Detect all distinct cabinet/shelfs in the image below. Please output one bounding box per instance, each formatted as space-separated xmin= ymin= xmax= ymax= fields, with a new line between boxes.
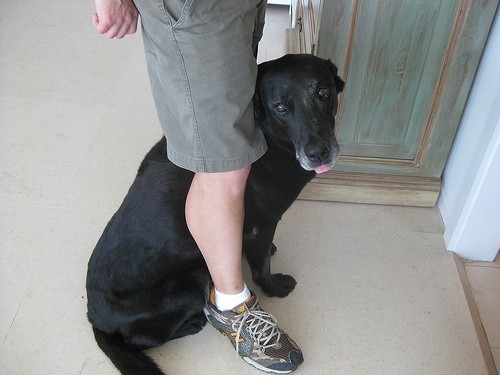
xmin=283 ymin=0 xmax=500 ymax=210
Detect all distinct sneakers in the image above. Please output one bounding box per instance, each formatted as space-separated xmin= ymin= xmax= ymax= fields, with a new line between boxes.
xmin=204 ymin=286 xmax=305 ymax=374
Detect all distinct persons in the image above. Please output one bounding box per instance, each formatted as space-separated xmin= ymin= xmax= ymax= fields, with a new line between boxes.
xmin=92 ymin=0 xmax=304 ymax=374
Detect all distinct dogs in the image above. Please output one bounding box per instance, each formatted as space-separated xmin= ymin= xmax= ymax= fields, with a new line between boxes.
xmin=85 ymin=53 xmax=347 ymax=375
